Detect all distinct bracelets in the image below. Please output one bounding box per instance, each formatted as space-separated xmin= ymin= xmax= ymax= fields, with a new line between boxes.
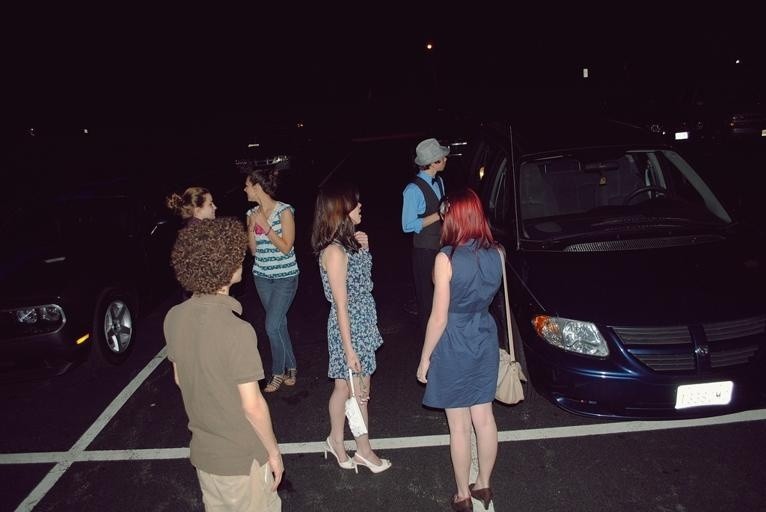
xmin=264 ymin=226 xmax=272 ymax=236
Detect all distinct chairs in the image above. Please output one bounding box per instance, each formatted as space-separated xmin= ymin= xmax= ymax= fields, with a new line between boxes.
xmin=519 ymin=154 xmax=650 ymax=221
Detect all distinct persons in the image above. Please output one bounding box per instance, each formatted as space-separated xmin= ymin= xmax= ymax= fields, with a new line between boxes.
xmin=160 ymin=187 xmax=218 ymax=303
xmin=243 ymin=170 xmax=301 ymax=394
xmin=162 ymin=218 xmax=285 ymax=512
xmin=401 ymin=137 xmax=451 ymax=366
xmin=310 ymin=177 xmax=392 ymax=476
xmin=415 ymin=188 xmax=507 ymax=512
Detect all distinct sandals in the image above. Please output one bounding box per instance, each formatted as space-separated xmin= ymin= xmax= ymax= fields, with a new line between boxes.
xmin=263 ymin=374 xmax=286 ymax=392
xmin=285 ymin=369 xmax=297 ymax=384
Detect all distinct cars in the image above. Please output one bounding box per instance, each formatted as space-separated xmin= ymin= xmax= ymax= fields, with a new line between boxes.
xmin=702 ymin=84 xmax=766 ymax=139
xmin=226 ymin=108 xmax=310 ymax=182
xmin=469 ymin=123 xmax=766 ymax=422
xmin=1 ymin=191 xmax=174 ymax=368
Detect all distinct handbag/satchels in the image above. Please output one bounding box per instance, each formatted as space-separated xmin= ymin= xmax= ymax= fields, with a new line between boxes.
xmin=495 ymin=349 xmax=527 ymax=404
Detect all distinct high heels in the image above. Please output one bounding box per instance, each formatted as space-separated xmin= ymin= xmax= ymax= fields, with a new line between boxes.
xmin=450 ymin=492 xmax=473 ymax=511
xmin=469 ymin=483 xmax=494 ymax=510
xmin=321 ymin=436 xmax=357 ymax=471
xmin=352 ymin=449 xmax=392 ymax=474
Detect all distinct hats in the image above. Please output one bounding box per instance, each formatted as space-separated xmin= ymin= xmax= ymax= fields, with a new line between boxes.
xmin=414 ymin=138 xmax=451 ymax=167
